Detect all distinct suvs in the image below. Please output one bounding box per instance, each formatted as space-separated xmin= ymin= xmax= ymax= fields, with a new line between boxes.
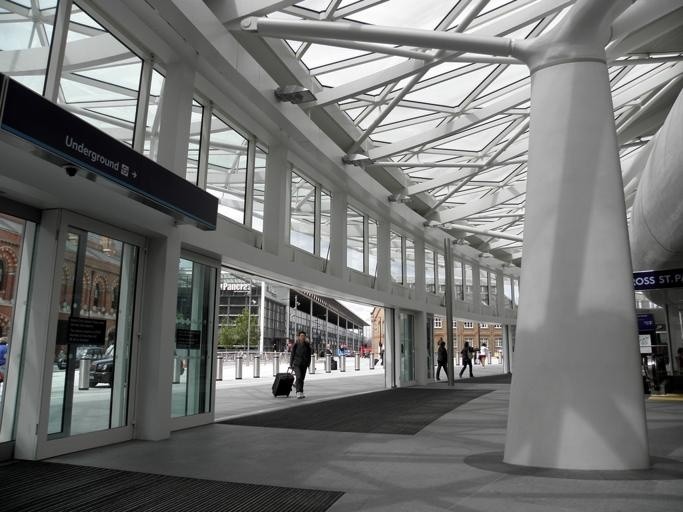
xmin=89 ymin=344 xmax=116 ymax=387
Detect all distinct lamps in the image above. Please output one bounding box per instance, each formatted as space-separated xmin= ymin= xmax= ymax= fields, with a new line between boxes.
xmin=340 ymin=154 xmax=373 ymax=168
xmin=502 ymin=261 xmax=515 ymax=268
xmin=423 ymin=220 xmax=443 ymax=229
xmin=387 ymin=192 xmax=412 ymax=206
xmin=452 ymin=238 xmax=471 ymax=246
xmin=477 ymin=252 xmax=495 ymax=262
xmin=271 ymin=83 xmax=317 ymax=107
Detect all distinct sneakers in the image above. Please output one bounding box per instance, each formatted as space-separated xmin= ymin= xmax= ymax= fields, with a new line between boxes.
xmin=435 ymin=377 xmax=441 ymax=381
xmin=459 ymin=373 xmax=462 ymax=378
xmin=469 ymin=374 xmax=474 ymax=378
xmin=296 ymin=392 xmax=306 ymax=399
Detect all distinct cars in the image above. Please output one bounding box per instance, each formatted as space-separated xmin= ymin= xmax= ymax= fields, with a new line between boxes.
xmin=345 ymin=349 xmax=355 ymax=356
xmin=494 ymin=347 xmax=503 ymax=358
xmin=56 ymin=345 xmax=106 ymax=370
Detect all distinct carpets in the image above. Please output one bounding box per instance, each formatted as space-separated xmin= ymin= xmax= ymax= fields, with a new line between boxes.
xmin=437 ymin=372 xmax=513 ymax=386
xmin=210 ymin=386 xmax=492 ymax=437
xmin=0 ymin=458 xmax=346 ymax=512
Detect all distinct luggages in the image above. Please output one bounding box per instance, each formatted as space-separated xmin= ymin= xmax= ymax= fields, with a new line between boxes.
xmin=324 ymin=360 xmax=337 ymax=370
xmin=372 ymin=359 xmax=379 ymax=365
xmin=271 ymin=372 xmax=294 ymax=398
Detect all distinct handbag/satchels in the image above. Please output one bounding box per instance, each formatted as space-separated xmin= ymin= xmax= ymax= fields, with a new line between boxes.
xmin=468 ymin=347 xmax=473 ymax=360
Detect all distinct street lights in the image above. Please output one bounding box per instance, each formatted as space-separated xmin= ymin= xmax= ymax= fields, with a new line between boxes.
xmin=247 ymin=298 xmax=257 ymax=357
xmin=370 ymin=315 xmax=381 ymax=355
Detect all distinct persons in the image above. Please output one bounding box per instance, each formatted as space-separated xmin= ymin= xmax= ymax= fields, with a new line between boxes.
xmin=435 ymin=337 xmax=490 ymax=381
xmin=57 ymin=349 xmax=66 ymax=359
xmin=0 ymin=336 xmax=8 ymax=383
xmin=675 ymin=348 xmax=683 ymax=378
xmin=323 ymin=341 xmax=387 ymax=366
xmin=273 ymin=340 xmax=294 ymax=353
xmin=289 ymin=331 xmax=311 ymax=399
xmin=642 ymin=356 xmax=652 ymax=394
